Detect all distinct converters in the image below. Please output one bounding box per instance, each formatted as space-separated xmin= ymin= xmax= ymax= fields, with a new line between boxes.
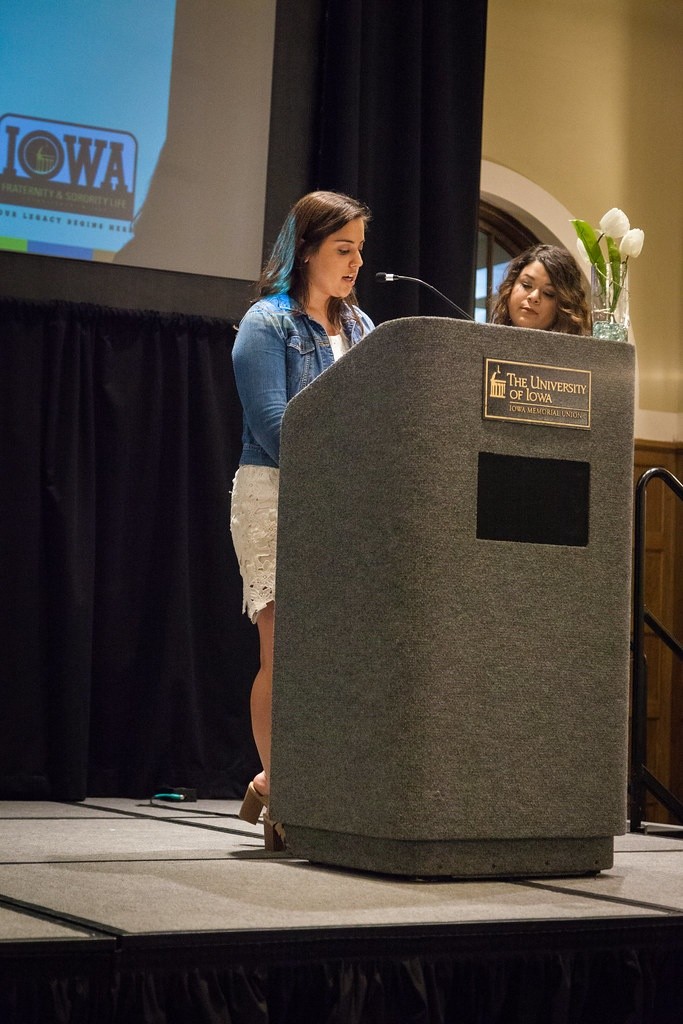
xmin=158 ymin=786 xmax=197 ymax=802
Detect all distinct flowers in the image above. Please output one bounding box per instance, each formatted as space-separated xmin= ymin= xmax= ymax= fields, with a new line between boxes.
xmin=569 ymin=208 xmax=645 ymax=319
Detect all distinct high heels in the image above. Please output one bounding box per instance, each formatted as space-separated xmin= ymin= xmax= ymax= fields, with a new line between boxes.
xmin=263 ymin=813 xmax=283 ymax=852
xmin=238 ymin=781 xmax=269 ymax=825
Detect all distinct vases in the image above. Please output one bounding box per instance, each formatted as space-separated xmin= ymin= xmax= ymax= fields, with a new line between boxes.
xmin=590 ymin=262 xmax=630 ymax=342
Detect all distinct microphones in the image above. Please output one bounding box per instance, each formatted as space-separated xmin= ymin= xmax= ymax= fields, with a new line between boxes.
xmin=376 ymin=273 xmax=475 ymax=321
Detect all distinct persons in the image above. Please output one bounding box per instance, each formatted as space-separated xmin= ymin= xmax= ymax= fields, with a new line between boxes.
xmin=490 ymin=243 xmax=593 ymax=338
xmin=230 ymin=190 xmax=374 ymax=855
